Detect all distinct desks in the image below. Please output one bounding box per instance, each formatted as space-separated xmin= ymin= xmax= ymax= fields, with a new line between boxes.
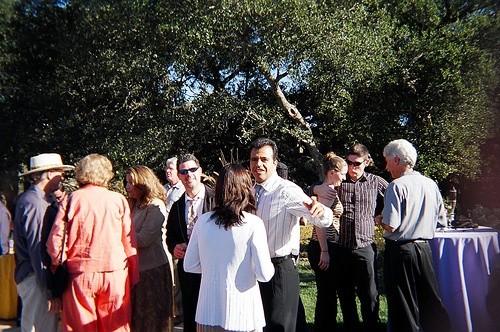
xmin=431 ymin=226 xmax=500 ymax=332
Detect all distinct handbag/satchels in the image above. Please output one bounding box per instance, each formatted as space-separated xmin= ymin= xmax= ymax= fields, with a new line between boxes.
xmin=46 ymin=265 xmax=70 ymax=296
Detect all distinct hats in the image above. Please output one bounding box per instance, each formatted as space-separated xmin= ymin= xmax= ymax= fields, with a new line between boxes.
xmin=19 ymin=154 xmax=75 ymax=176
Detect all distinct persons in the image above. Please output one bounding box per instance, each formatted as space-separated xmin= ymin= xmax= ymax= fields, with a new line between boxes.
xmin=304 ymin=143 xmax=390 ymax=332
xmin=307 ymin=152 xmax=348 ymax=332
xmin=0 ymin=138 xmax=333 ymax=332
xmin=375 ymin=138 xmax=450 ymax=332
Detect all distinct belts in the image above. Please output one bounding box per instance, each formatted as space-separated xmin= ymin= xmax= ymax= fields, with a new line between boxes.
xmin=272 ymin=254 xmax=293 ymax=265
xmin=405 ymin=239 xmax=427 ymax=243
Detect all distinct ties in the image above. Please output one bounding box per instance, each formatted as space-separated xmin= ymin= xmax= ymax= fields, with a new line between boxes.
xmin=187 ymin=200 xmax=198 ymax=240
xmin=254 ymin=184 xmax=263 ymax=210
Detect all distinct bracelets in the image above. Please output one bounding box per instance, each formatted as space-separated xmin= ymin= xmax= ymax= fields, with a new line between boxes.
xmin=321 ymin=249 xmax=328 ymax=252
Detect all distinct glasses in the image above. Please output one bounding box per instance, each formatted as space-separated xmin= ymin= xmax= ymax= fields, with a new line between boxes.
xmin=346 ymin=159 xmax=365 ymax=166
xmin=178 ymin=166 xmax=200 ymax=175
